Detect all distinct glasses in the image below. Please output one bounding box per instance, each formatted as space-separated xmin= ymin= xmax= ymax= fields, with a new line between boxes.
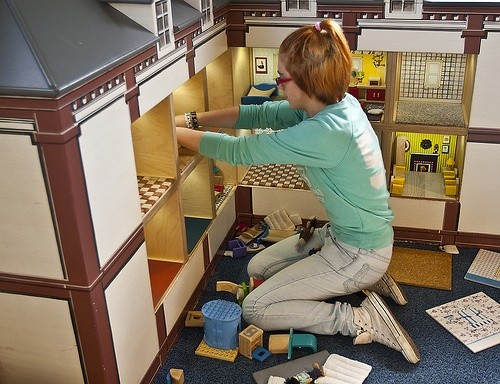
xmin=276 ymin=76 xmax=292 ymax=86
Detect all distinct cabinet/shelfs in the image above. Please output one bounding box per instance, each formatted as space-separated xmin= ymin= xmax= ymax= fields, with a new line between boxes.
xmin=347 ymin=87 xmax=359 ymax=101
xmin=366 ymin=88 xmax=385 ymax=102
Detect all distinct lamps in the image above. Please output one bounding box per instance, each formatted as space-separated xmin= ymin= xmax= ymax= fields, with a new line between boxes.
xmin=446 ymin=157 xmax=455 ymax=171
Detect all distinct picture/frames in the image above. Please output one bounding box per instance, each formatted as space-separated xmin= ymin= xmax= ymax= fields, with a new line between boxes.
xmin=442 ymin=135 xmax=451 ymax=144
xmin=442 ymin=145 xmax=449 ymax=154
xmin=351 ymin=56 xmax=363 ymax=73
xmin=255 ymin=57 xmax=268 ymax=74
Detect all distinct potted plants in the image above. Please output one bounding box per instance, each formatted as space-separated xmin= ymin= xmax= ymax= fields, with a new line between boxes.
xmin=355 ymin=70 xmax=364 ymax=83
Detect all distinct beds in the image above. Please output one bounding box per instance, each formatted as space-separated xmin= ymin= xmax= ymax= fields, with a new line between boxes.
xmin=241 ymin=82 xmax=279 ymax=106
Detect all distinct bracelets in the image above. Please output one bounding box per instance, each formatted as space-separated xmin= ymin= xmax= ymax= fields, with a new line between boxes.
xmin=184 ymin=112 xmax=198 ymax=129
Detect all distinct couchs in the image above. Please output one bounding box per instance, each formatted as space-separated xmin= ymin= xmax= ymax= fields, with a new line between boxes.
xmin=444 ymin=180 xmax=458 ymax=196
xmin=444 ymin=170 xmax=456 ymax=186
xmin=394 ymin=166 xmax=406 ymax=180
xmin=390 ymin=179 xmax=404 ymax=195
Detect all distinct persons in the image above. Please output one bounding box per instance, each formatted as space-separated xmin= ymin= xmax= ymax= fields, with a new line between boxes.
xmin=174 ymin=19 xmax=420 ymax=363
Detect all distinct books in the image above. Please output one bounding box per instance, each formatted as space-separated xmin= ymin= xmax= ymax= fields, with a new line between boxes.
xmin=426 ymin=292 xmax=500 ymax=353
xmin=464 ymin=249 xmax=500 ymax=289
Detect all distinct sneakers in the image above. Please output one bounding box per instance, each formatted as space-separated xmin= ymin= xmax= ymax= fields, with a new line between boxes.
xmin=362 ymin=271 xmax=408 ymax=305
xmin=352 ymin=292 xmax=421 ymax=363
